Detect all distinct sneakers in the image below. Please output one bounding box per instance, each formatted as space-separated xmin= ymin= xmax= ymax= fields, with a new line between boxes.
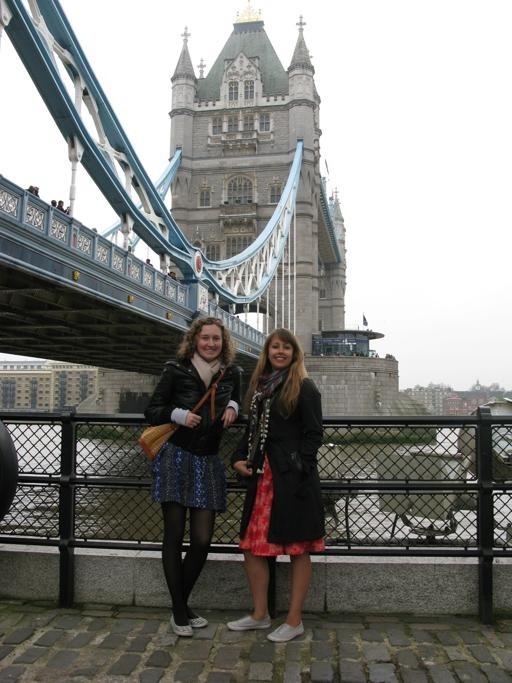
xmin=267 ymin=621 xmax=305 ymax=643
xmin=227 ymin=615 xmax=271 ymax=632
xmin=188 ymin=615 xmax=209 ymax=628
xmin=169 ymin=613 xmax=194 ymax=636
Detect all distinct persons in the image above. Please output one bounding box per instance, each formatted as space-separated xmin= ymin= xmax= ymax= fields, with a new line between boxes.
xmin=168 ymin=271 xmax=177 ymax=280
xmin=230 ymin=328 xmax=326 ymax=642
xmin=144 ymin=316 xmax=244 ymax=638
xmin=145 ymin=258 xmax=153 ymax=266
xmin=333 ymin=349 xmax=392 ymax=358
xmin=25 ymin=185 xmax=70 ymax=214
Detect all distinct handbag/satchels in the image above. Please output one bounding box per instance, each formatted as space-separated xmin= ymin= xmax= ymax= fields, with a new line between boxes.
xmin=137 ymin=421 xmax=177 ymax=460
